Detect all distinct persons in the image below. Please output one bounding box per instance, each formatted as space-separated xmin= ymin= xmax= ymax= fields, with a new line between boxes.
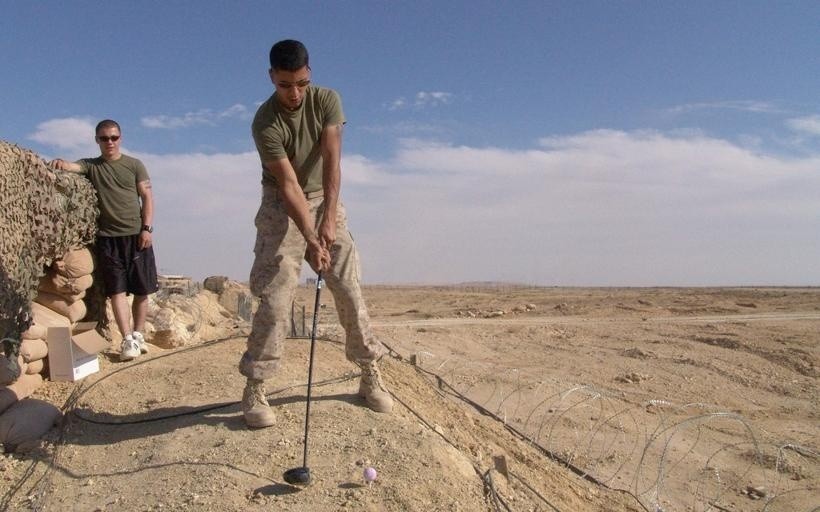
xmin=45 ymin=118 xmax=159 ymax=362
xmin=238 ymin=39 xmax=396 ymax=428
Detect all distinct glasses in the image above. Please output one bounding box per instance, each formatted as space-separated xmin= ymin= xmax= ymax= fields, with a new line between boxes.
xmin=270 ymin=65 xmax=312 ymax=88
xmin=97 ymin=135 xmax=120 ymax=142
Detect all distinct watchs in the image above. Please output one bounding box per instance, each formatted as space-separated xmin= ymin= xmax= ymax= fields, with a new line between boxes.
xmin=141 ymin=224 xmax=153 ymax=233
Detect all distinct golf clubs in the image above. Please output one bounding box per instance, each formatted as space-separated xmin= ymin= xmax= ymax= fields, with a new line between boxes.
xmin=284 ymin=270 xmax=322 ymax=487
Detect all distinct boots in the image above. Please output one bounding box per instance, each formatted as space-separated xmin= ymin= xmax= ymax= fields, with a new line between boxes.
xmin=242 ymin=380 xmax=276 ymax=429
xmin=359 ymin=362 xmax=394 ymax=413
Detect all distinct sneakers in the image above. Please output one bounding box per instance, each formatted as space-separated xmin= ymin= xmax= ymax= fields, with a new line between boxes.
xmin=132 ymin=331 xmax=148 ymax=353
xmin=119 ymin=336 xmax=141 ymax=362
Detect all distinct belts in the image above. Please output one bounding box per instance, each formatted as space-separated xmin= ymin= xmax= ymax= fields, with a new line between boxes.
xmin=263 ymin=184 xmax=325 ymax=201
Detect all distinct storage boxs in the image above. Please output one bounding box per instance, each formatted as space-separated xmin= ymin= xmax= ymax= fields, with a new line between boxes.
xmin=46 ymin=320 xmax=111 ymax=382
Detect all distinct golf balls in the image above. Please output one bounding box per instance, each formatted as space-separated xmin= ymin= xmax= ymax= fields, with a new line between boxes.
xmin=364 ymin=468 xmax=376 ymax=480
